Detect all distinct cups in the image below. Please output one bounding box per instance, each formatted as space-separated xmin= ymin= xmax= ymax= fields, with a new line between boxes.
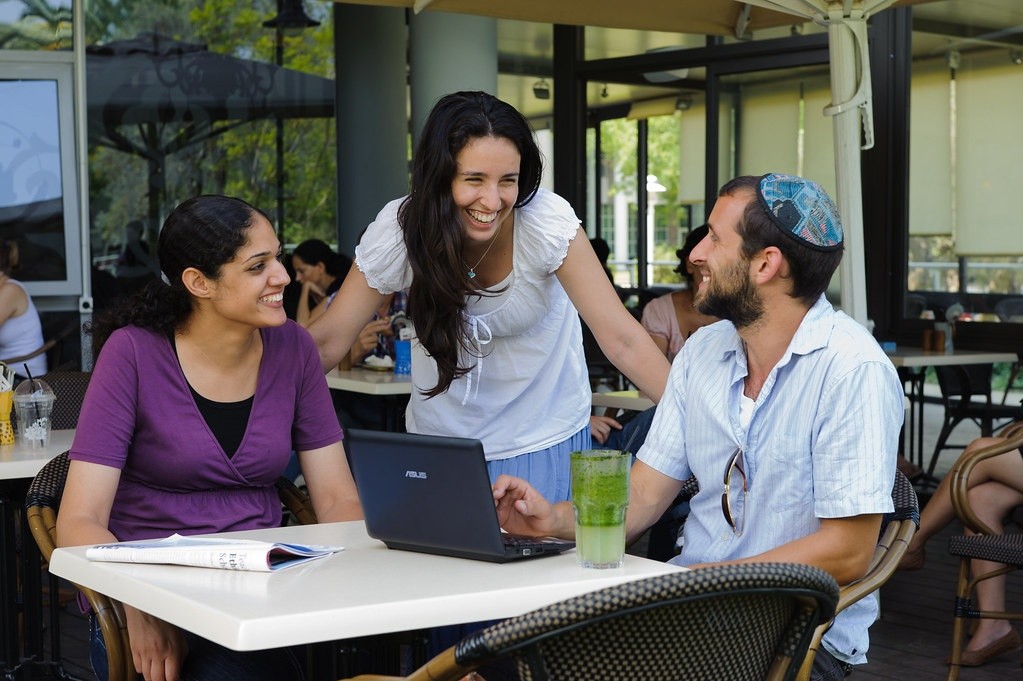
xmin=571 ymin=449 xmax=630 ymax=571
xmin=11 ymin=379 xmax=55 ymax=452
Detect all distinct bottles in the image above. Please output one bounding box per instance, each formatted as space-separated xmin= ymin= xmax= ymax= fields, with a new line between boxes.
xmin=0 ymin=391 xmax=16 ymax=446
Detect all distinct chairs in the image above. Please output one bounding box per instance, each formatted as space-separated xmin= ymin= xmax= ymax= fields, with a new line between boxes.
xmin=0 ymin=286 xmax=1023 ymax=681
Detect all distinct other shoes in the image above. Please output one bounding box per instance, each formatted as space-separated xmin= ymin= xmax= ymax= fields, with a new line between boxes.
xmin=896 ymin=547 xmax=926 ymax=570
xmin=945 ymin=628 xmax=1021 ymax=666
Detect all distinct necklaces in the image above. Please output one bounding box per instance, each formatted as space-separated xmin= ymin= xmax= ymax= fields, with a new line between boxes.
xmin=463 ymin=226 xmax=501 ymax=279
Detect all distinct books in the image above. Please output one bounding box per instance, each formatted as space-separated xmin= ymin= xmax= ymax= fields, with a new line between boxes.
xmin=86 ymin=534 xmax=344 ymax=573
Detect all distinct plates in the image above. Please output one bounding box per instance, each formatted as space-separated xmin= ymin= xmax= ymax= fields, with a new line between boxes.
xmin=357 ymin=362 xmax=395 ymax=371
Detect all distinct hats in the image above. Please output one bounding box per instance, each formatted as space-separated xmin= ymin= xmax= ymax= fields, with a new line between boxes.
xmin=756 ymin=173 xmax=844 ymax=251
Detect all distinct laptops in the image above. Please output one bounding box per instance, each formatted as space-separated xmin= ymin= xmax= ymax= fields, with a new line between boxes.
xmin=347 ymin=429 xmax=576 ymax=563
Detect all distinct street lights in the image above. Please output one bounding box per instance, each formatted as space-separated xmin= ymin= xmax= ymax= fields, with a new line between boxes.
xmin=647 ymin=174 xmax=667 ymax=286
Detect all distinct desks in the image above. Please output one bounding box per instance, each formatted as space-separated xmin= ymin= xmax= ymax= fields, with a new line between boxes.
xmin=0 ymin=428 xmax=77 ymax=482
xmin=883 ymin=352 xmax=1018 ymax=504
xmin=51 ymin=519 xmax=691 ymax=681
xmin=326 ymin=366 xmax=412 ymax=395
xmin=591 ymin=390 xmax=655 ymax=411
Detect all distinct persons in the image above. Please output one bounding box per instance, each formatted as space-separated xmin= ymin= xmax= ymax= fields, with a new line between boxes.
xmin=895 ymin=435 xmax=1023 ymax=667
xmin=639 ymin=223 xmax=720 ymax=361
xmin=292 ymin=227 xmax=409 ymax=371
xmin=0 ymin=239 xmax=48 ymax=378
xmin=55 ymin=195 xmax=378 ymax=681
xmin=589 ymin=405 xmax=693 ymax=561
xmin=590 ymin=238 xmax=614 ymax=286
xmin=492 ymin=170 xmax=903 ymax=681
xmin=304 ymin=90 xmax=673 ymax=503
xmin=117 ymin=220 xmax=150 ymax=281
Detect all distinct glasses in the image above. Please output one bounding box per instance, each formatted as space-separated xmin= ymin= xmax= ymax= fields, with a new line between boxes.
xmin=721 ymin=448 xmax=747 ymax=537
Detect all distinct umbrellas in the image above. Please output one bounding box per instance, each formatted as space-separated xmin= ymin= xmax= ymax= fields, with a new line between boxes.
xmin=88 ymin=31 xmax=337 ymax=274
